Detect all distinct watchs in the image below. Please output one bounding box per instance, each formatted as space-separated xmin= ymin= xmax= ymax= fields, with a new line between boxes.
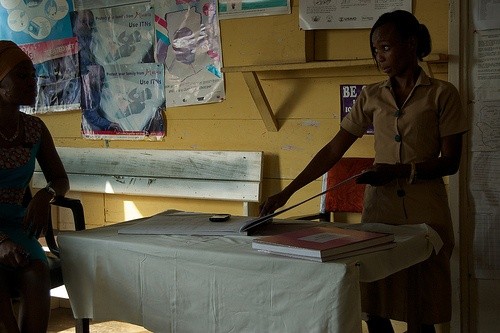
xmin=44 ymin=186 xmax=57 ymax=203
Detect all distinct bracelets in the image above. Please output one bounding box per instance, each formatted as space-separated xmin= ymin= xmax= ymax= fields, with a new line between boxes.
xmin=0 ymin=231 xmax=9 ymax=244
xmin=405 ymin=160 xmax=417 ymax=185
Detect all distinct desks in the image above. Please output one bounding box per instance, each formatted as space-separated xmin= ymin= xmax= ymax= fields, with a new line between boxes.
xmin=58 ymin=209 xmax=443 ymax=333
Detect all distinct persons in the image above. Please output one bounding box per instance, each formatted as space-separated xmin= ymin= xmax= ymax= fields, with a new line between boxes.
xmin=0 ymin=39 xmax=70 ymax=333
xmin=259 ymin=9 xmax=464 ymax=333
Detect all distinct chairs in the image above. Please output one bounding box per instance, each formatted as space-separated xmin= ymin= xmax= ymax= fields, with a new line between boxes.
xmin=288 ymin=158 xmax=375 ymax=223
xmin=0 ymin=185 xmax=153 ymax=333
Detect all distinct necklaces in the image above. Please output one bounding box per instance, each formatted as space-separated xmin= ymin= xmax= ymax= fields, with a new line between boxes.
xmin=0 ymin=111 xmax=21 ymax=141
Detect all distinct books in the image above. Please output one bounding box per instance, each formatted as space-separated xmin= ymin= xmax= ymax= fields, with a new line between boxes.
xmin=252 ymin=225 xmax=396 ymax=262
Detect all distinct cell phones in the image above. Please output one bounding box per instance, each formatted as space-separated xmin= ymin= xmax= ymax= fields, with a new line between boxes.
xmin=209 ymin=214 xmax=230 ymax=222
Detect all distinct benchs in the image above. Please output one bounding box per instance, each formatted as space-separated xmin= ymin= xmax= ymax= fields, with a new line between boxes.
xmin=32 ymin=147 xmax=264 ymax=300
